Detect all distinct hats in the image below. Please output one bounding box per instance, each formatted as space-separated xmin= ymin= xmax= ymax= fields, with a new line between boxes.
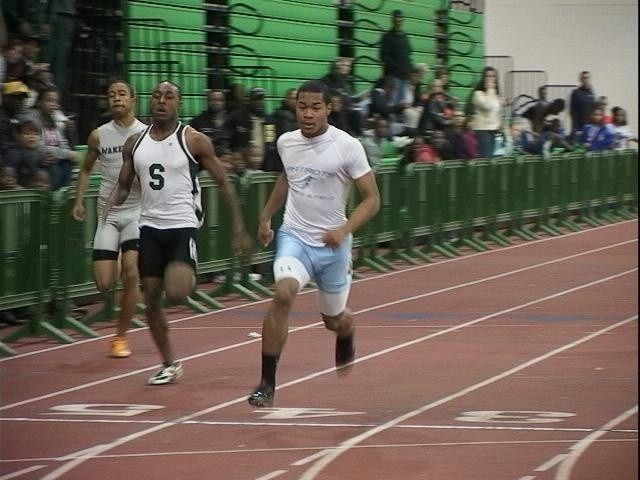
xmin=3 ymin=81 xmax=33 ymax=98
xmin=24 ymin=33 xmax=42 ymax=41
xmin=250 ymin=86 xmax=267 ymax=96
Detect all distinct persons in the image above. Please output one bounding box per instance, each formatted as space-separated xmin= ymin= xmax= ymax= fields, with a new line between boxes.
xmin=26 ymin=166 xmax=55 ymax=192
xmin=540 ymin=118 xmax=576 ymax=152
xmin=466 ymin=67 xmax=504 ymax=157
xmin=604 ymin=106 xmax=638 ymax=150
xmin=6 ymin=33 xmax=49 ymax=82
xmin=458 ymin=117 xmax=481 ymax=159
xmin=418 ymin=87 xmax=464 ymax=143
xmin=69 ymin=79 xmax=150 ymax=358
xmin=514 ymin=98 xmax=565 ymax=154
xmin=187 ymin=88 xmax=235 ymax=157
xmin=0 ymin=40 xmax=26 ymax=83
xmin=34 ymin=70 xmax=58 ymax=94
xmin=598 ymin=96 xmax=608 ymax=110
xmin=321 ymin=57 xmax=385 ymax=135
xmin=328 ymin=95 xmax=346 ymax=131
xmin=0 ymin=81 xmax=42 ymax=151
xmin=230 ymin=147 xmax=264 ymax=176
xmin=273 ymin=87 xmax=298 ymax=173
xmin=381 ymin=10 xmax=413 ymax=109
xmin=501 ymin=123 xmax=532 ymax=156
xmin=28 ymin=86 xmax=71 ymax=148
xmin=101 ymin=79 xmax=256 ymax=386
xmin=223 ymin=85 xmax=271 ymax=170
xmin=419 ymin=78 xmax=444 ymax=104
xmin=416 ymin=129 xmax=455 ymax=163
xmin=248 ymin=80 xmax=381 ymax=408
xmin=0 ymin=164 xmax=26 ymax=191
xmin=570 ymin=71 xmax=596 ymax=145
xmin=2 ymin=120 xmax=83 ymax=191
xmin=573 ymin=107 xmax=615 ymax=153
xmin=539 ymin=88 xmax=547 ymax=99
xmin=196 ymin=147 xmax=234 ymax=178
xmin=360 ymin=117 xmax=394 ymax=166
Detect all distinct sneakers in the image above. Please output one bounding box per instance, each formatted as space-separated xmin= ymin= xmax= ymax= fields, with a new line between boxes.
xmin=336 ymin=334 xmax=355 ymax=367
xmin=148 ymin=360 xmax=184 ymax=385
xmin=247 ymin=385 xmax=275 ymax=408
xmin=107 ymin=337 xmax=132 ymax=357
